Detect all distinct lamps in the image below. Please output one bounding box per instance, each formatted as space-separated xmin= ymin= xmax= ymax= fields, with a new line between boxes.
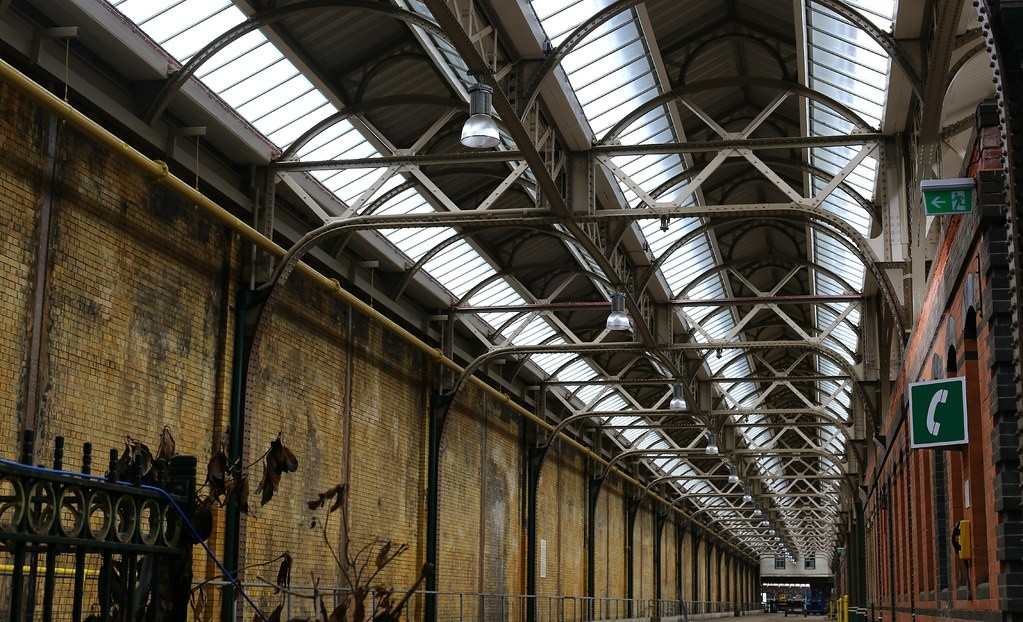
xmin=670 ymin=376 xmax=686 ymax=410
xmin=754 ymin=503 xmax=797 ymax=565
xmin=460 ymin=68 xmax=501 ymax=149
xmin=728 ymin=466 xmax=738 ymax=483
xmin=743 ymin=487 xmax=751 ymax=501
xmin=606 ymin=285 xmax=630 ymax=330
xmin=706 ymin=434 xmax=718 ymax=454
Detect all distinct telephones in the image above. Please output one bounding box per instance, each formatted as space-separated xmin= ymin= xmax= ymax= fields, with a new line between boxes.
xmin=952 ymin=520 xmax=970 ymax=560
